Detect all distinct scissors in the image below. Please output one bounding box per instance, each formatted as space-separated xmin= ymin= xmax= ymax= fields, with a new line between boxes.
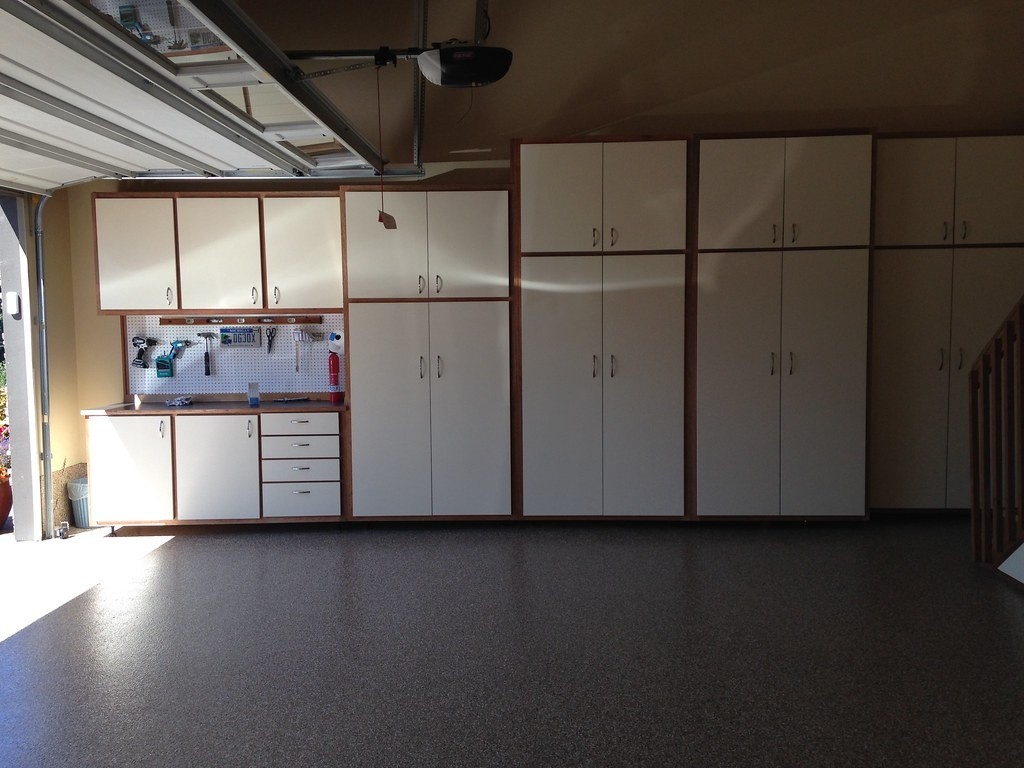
xmin=266 ymin=328 xmax=276 ymax=354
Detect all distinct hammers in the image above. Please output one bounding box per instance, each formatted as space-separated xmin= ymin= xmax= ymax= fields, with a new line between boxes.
xmin=197 ymin=332 xmax=218 ymax=375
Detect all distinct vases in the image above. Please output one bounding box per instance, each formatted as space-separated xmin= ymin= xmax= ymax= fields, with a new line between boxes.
xmin=0 ymin=481 xmax=12 ymax=529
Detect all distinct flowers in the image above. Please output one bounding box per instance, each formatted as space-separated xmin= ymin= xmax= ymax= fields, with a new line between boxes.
xmin=0 ymin=460 xmax=11 ymax=485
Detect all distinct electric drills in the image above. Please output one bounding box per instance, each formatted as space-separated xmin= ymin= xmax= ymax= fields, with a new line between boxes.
xmin=169 ymin=340 xmax=191 ymax=359
xmin=132 ymin=336 xmax=158 ymax=368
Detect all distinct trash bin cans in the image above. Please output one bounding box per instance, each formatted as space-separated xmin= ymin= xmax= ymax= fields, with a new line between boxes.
xmin=67 ymin=477 xmax=104 ymax=530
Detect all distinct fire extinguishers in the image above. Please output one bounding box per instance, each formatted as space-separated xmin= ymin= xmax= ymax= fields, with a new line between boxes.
xmin=328 ymin=349 xmax=340 ymax=401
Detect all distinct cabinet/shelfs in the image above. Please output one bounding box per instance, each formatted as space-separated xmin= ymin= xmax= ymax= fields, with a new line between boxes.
xmin=175 ymin=191 xmax=344 ymax=315
xmin=693 ymin=130 xmax=872 ymax=521
xmin=510 ymin=137 xmax=694 ymax=521
xmin=338 ymin=180 xmax=512 ymax=521
xmin=84 ymin=415 xmax=175 ymax=528
xmin=257 ymin=412 xmax=343 ymax=523
xmin=91 ymin=191 xmax=180 ymax=316
xmin=866 ymin=135 xmax=1024 ymax=510
xmin=174 ymin=414 xmax=260 ymax=526
